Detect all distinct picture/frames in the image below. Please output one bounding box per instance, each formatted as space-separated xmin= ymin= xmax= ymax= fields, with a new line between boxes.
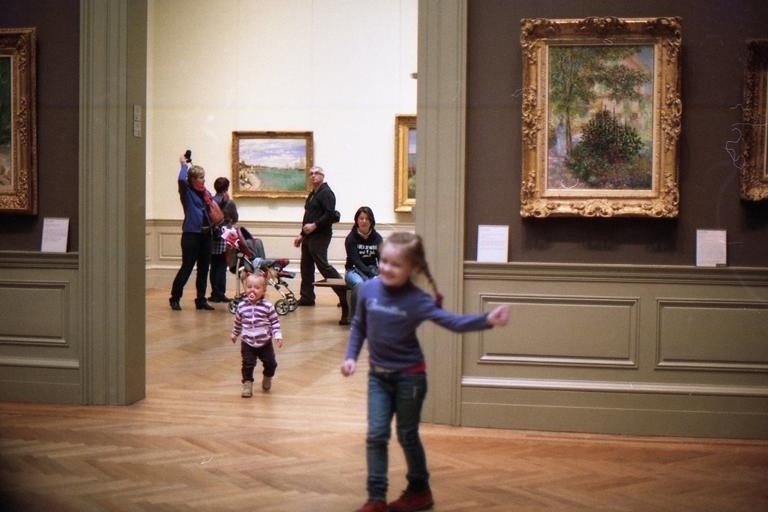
xmin=392 ymin=110 xmax=421 ymax=214
xmin=515 ymin=13 xmax=683 ymax=221
xmin=228 ymin=128 xmax=316 ymax=201
xmin=0 ymin=24 xmax=41 ymax=220
xmin=736 ymin=38 xmax=768 ymax=204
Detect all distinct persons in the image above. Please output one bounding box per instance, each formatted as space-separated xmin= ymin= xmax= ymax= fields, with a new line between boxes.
xmin=343 ymin=205 xmax=384 ymax=325
xmin=166 ymin=153 xmax=216 ymax=312
xmin=338 ymin=230 xmax=511 ymax=510
xmin=290 ymin=165 xmax=348 ymax=309
xmin=228 ymin=273 xmax=284 ymax=399
xmin=206 ymin=177 xmax=238 ymax=303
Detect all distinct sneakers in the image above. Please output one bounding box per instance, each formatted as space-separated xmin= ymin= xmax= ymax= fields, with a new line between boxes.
xmin=355 ymin=500 xmax=387 ymax=512
xmin=241 ymin=381 xmax=252 ymax=396
xmin=389 ymin=492 xmax=433 ymax=511
xmin=262 ymin=375 xmax=271 ymax=390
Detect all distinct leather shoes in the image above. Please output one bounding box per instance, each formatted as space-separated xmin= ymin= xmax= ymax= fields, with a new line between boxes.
xmin=208 ymin=296 xmax=233 ymax=302
xmin=196 ymin=299 xmax=214 ymax=310
xmin=297 ymin=299 xmax=315 ymax=306
xmin=170 ymin=298 xmax=181 ymax=310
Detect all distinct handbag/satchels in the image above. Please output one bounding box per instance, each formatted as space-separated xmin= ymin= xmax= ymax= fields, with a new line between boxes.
xmin=208 ymin=199 xmax=224 ymax=226
xmin=332 ymin=211 xmax=340 ymax=222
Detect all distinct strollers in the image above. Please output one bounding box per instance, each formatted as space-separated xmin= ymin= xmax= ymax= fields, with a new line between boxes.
xmin=220 ymin=219 xmax=301 ymax=317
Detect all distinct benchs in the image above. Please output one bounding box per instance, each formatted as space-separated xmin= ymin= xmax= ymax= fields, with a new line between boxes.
xmin=310 ymin=276 xmax=353 ymax=327
